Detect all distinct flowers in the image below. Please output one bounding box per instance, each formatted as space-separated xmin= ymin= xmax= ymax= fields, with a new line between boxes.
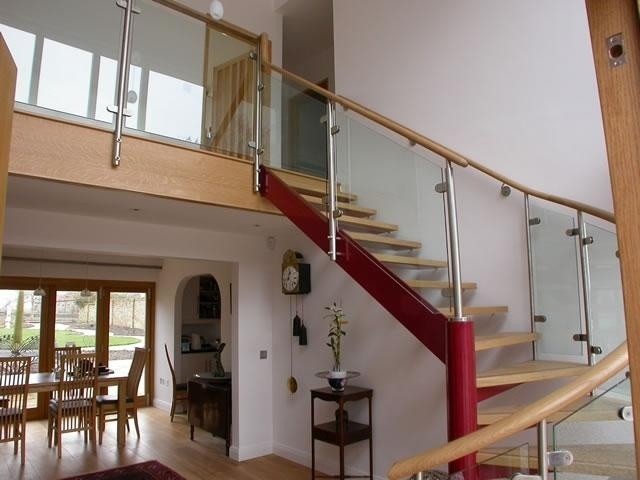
xmin=0 ymin=334 xmax=39 ymax=355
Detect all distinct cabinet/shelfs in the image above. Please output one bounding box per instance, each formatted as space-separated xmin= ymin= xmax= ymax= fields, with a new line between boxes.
xmin=181 ymin=319 xmax=221 ymax=390
xmin=191 ymin=275 xmax=221 ymax=319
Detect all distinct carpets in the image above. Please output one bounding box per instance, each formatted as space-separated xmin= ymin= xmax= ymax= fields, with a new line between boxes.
xmin=55 ymin=460 xmax=186 ymax=480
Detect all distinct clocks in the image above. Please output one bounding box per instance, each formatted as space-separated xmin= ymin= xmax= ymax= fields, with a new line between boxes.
xmin=281 ymin=249 xmax=311 ymax=393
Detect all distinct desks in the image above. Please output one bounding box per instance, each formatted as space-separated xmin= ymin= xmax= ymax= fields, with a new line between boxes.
xmin=311 ymin=385 xmax=373 ymax=480
xmin=186 ymin=379 xmax=231 ymax=457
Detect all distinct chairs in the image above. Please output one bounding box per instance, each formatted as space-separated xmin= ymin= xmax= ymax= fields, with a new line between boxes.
xmin=1 ymin=347 xmax=148 ymax=459
xmin=165 ymin=343 xmax=202 ymax=423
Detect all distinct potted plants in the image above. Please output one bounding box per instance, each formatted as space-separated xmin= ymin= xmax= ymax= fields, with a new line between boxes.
xmin=316 ymin=297 xmax=360 ymax=393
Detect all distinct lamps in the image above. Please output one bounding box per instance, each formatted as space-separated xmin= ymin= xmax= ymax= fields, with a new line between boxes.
xmin=81 ymin=262 xmax=91 ymax=297
xmin=33 ymin=259 xmax=46 ymax=296
